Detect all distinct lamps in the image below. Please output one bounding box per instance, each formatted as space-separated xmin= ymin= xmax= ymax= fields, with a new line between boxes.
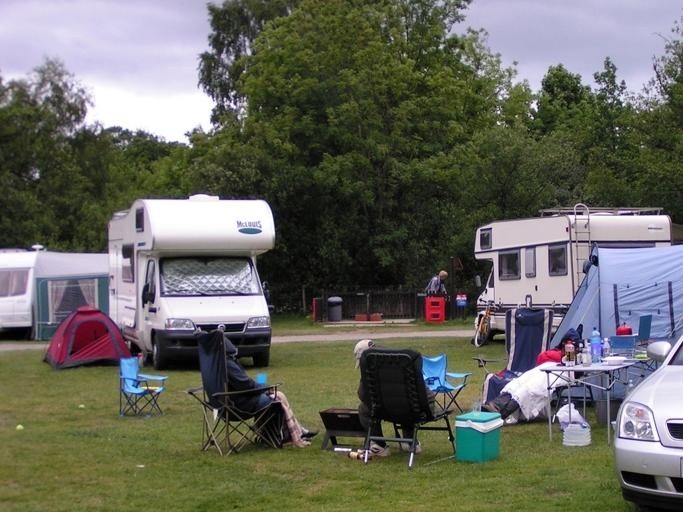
xmin=482 ymin=306 xmax=553 ymax=406
xmin=423 ymin=355 xmax=471 ymax=413
xmin=361 ymin=348 xmax=454 ymax=467
xmin=552 ymin=324 xmax=595 ymax=424
xmin=118 ymin=356 xmax=167 ymax=419
xmin=188 ymin=331 xmax=293 ymax=455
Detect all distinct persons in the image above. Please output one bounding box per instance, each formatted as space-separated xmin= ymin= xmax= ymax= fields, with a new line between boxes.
xmin=345 ymin=339 xmax=435 ymax=462
xmin=454 ymin=288 xmax=469 ymax=320
xmin=482 ymin=327 xmax=580 ymax=422
xmin=225 ymin=338 xmax=319 ymax=442
xmin=420 ymin=271 xmax=448 ymax=294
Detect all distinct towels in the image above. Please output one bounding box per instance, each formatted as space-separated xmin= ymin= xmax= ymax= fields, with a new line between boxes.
xmin=327 ymin=297 xmax=343 ymax=321
xmin=455 ymin=411 xmax=504 ymax=462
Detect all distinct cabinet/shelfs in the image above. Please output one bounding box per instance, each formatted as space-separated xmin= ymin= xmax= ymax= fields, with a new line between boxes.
xmin=564 ymin=327 xmax=610 ymax=367
xmin=347 ymin=451 xmax=364 ymax=461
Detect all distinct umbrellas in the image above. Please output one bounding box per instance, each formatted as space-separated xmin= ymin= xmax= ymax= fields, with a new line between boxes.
xmin=301 ymin=430 xmax=319 ymax=439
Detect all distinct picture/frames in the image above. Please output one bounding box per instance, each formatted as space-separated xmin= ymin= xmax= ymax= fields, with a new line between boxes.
xmin=540 ymin=356 xmax=658 ymax=446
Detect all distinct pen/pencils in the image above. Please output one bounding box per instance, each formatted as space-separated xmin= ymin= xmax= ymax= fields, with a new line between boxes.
xmin=1 ymin=244 xmax=110 ymax=330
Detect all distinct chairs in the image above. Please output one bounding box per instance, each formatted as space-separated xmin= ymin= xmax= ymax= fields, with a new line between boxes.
xmin=357 ymin=444 xmax=391 ymax=456
xmin=481 ymin=405 xmax=498 ymax=411
xmin=401 ymin=439 xmax=422 ymax=455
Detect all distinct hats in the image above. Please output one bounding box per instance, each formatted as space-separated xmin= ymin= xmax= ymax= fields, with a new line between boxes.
xmin=614 ymin=325 xmax=683 ymax=505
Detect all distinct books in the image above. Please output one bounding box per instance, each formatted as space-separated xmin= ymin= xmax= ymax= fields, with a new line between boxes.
xmin=454 ymin=411 xmax=502 ymax=464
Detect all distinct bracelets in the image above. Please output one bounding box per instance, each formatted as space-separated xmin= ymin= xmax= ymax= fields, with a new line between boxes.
xmin=605 ymin=356 xmax=623 ymax=365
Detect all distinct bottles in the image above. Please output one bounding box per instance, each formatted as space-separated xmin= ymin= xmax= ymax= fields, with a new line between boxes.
xmin=354 ymin=340 xmax=376 ymax=369
